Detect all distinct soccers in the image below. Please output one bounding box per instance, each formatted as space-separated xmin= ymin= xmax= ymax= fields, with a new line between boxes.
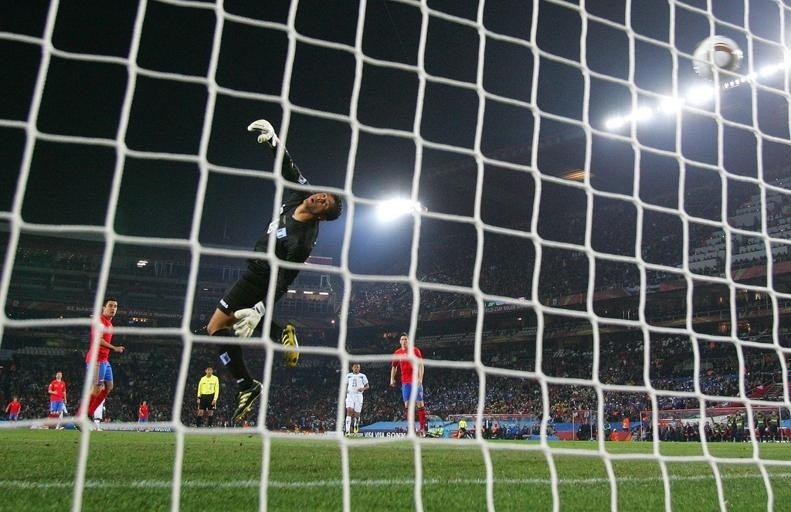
xmin=693 ymin=36 xmax=743 ymax=81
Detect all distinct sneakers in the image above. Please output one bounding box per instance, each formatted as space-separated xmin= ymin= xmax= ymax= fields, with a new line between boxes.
xmin=354 ymin=426 xmax=359 ymax=433
xmin=345 ymin=432 xmax=350 ymax=437
xmin=231 ymin=379 xmax=263 ymax=422
xmin=282 ymin=324 xmax=300 ymax=368
xmin=417 ymin=429 xmax=426 ymax=438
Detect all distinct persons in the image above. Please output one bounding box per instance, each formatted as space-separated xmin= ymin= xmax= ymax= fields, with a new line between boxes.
xmin=390 ymin=332 xmax=429 ymax=438
xmin=206 ymin=119 xmax=343 ymax=422
xmin=351 ymin=180 xmax=790 ymax=337
xmin=71 ymin=297 xmax=126 ymax=431
xmin=0 ymin=340 xmax=790 ymax=441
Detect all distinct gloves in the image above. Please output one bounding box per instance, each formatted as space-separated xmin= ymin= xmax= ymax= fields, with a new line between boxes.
xmin=247 ymin=118 xmax=278 ymax=149
xmin=234 ymin=301 xmax=266 ymax=338
